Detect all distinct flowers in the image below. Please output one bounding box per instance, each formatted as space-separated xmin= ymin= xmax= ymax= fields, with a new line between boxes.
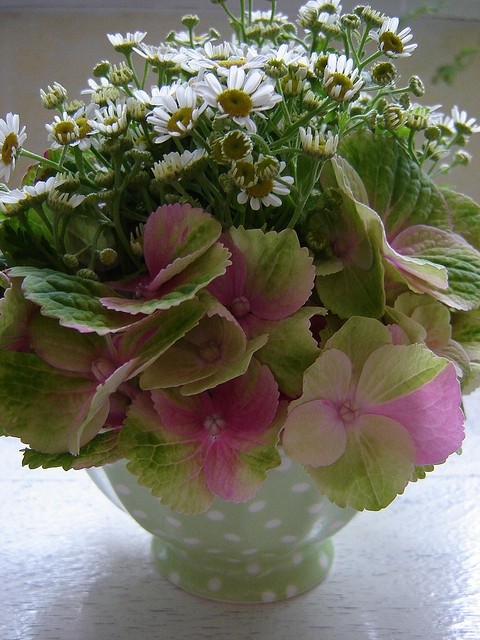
xmin=0 ymin=0 xmax=480 ymax=515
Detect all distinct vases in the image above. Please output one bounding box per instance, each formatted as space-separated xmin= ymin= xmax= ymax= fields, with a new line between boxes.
xmin=83 ymin=426 xmax=359 ymax=606
